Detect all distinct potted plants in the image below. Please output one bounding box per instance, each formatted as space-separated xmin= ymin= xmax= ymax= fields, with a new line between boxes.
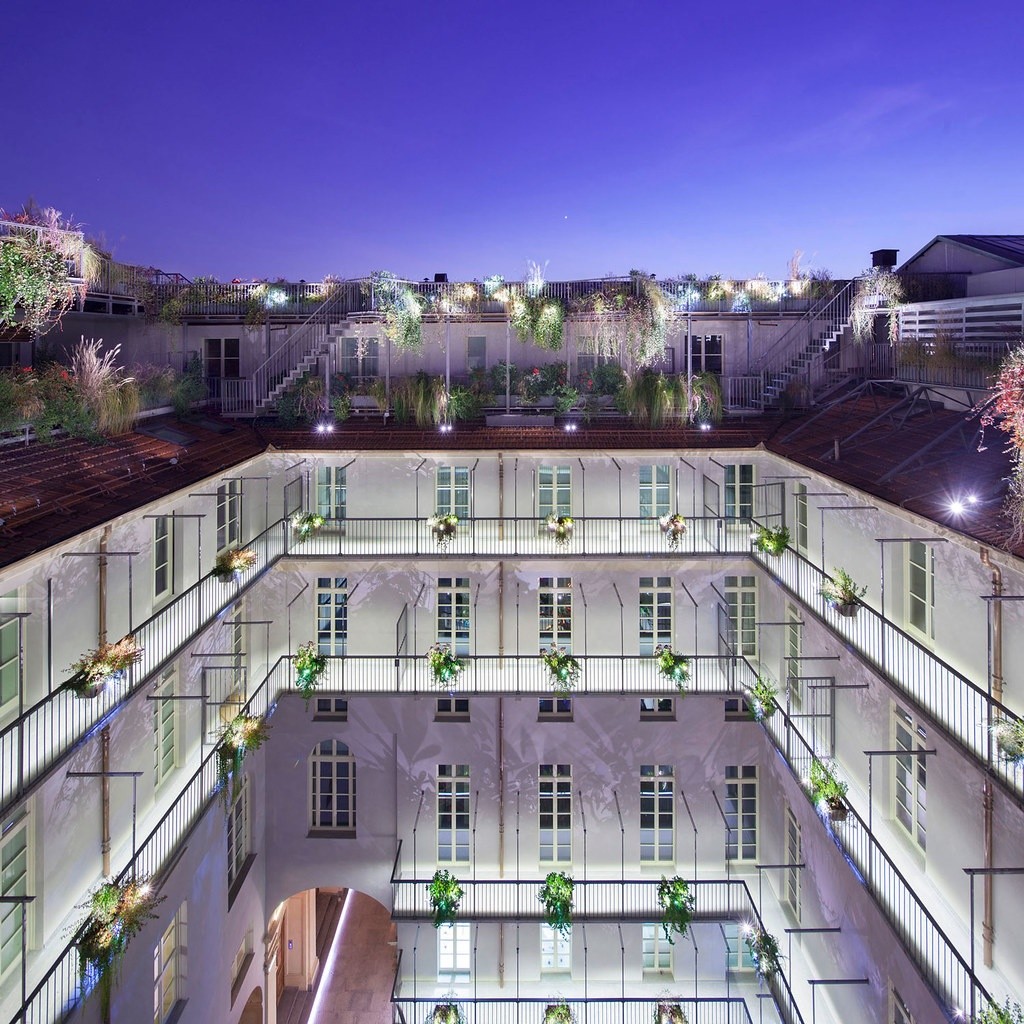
xmin=426 ymin=513 xmax=459 ymax=544
xmin=64 ymin=547 xmax=1024 ymax=1024
xmin=755 ymin=526 xmax=790 ymax=557
xmin=546 ymin=512 xmax=574 ymax=546
xmin=659 ymin=514 xmax=688 ymax=546
xmin=292 ymin=511 xmax=324 ymax=543
